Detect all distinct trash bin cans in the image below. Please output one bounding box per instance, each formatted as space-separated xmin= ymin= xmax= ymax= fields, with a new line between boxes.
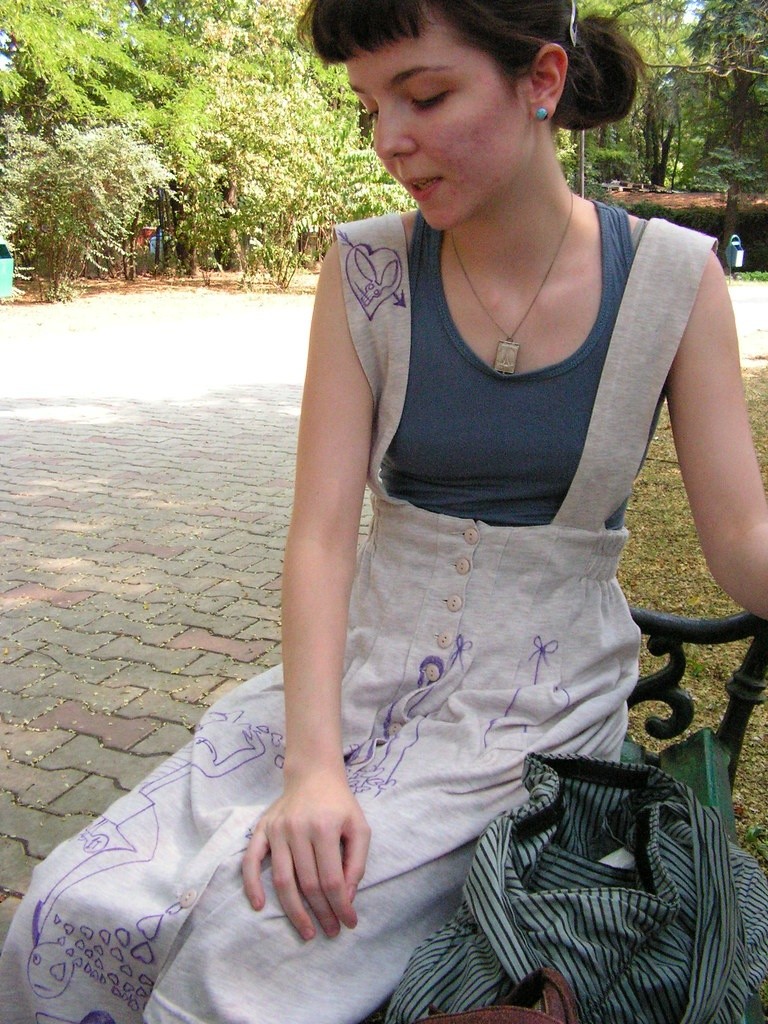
xmin=0 ymin=244 xmax=15 ymax=298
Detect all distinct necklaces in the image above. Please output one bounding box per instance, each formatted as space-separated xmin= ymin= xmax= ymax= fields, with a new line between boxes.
xmin=449 ymin=189 xmax=573 ymax=374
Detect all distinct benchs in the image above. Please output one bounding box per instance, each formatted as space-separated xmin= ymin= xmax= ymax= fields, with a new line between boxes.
xmin=618 ymin=606 xmax=767 ymax=839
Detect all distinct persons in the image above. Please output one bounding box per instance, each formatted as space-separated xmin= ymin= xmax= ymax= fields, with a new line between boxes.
xmin=0 ymin=0 xmax=766 ymax=1024
